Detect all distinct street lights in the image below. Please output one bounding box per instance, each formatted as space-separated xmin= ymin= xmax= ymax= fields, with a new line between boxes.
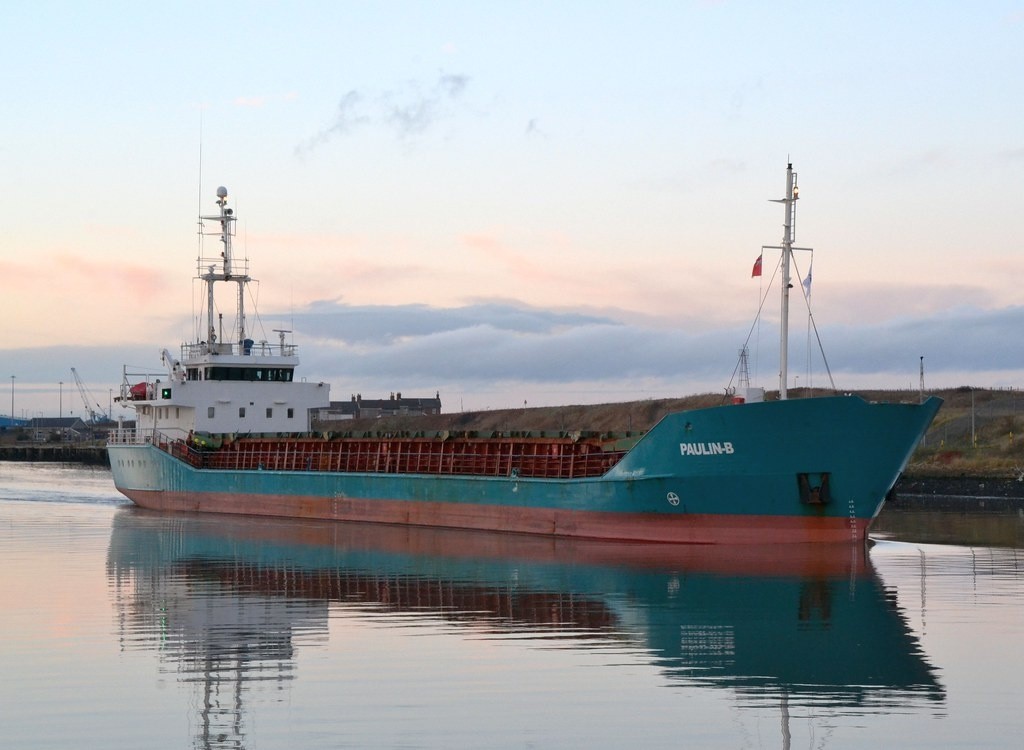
xmin=58 ymin=381 xmax=65 ymax=419
xmin=9 ymin=374 xmax=17 ymax=426
xmin=109 ymin=388 xmax=113 ymax=419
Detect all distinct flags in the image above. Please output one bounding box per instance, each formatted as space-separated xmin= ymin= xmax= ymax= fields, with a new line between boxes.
xmin=803 ymin=267 xmax=811 ymax=298
xmin=751 ymin=255 xmax=762 ymax=278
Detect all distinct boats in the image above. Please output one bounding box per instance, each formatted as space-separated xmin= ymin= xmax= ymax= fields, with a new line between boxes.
xmin=106 ymin=502 xmax=953 ymax=749
xmin=102 ymin=153 xmax=944 ymax=539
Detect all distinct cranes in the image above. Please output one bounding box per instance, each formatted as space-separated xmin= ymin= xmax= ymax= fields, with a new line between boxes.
xmin=69 ymin=368 xmax=107 ymax=424
